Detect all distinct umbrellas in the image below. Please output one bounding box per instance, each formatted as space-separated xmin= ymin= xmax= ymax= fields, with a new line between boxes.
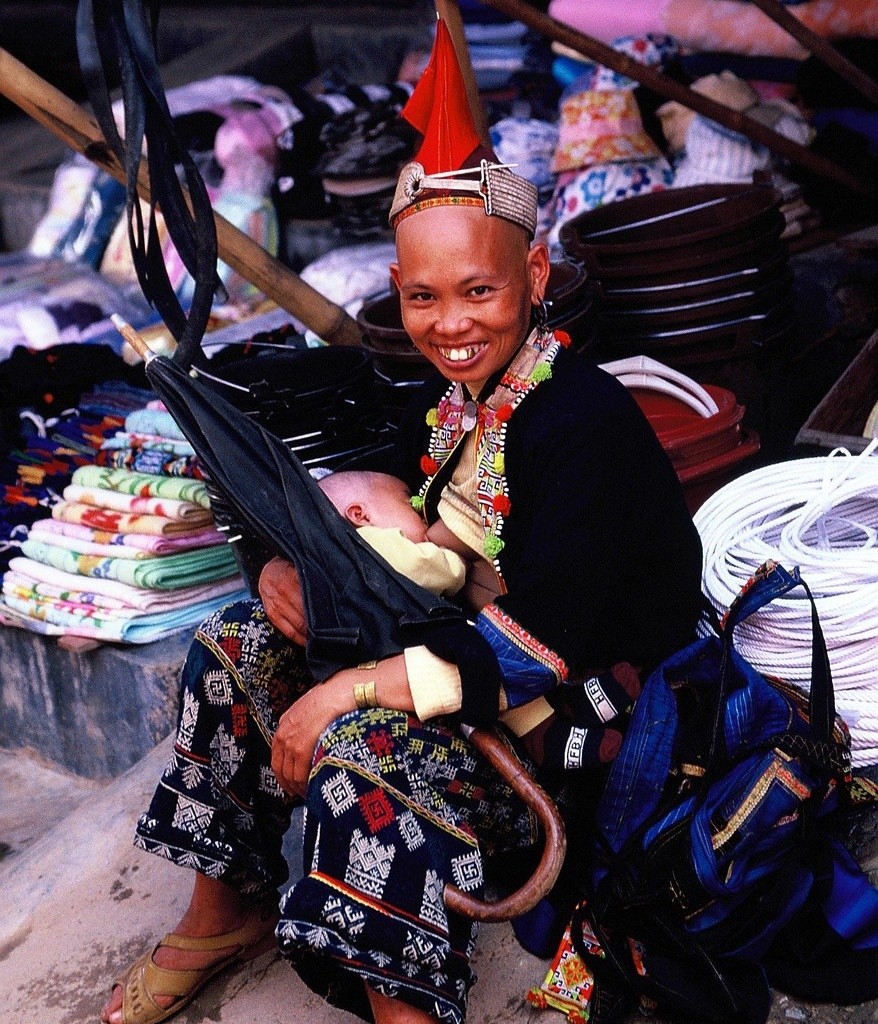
xmin=108 ymin=314 xmax=566 ymax=923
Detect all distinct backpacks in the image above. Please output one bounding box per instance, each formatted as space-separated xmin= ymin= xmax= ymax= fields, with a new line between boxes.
xmin=571 ymin=556 xmax=851 ymax=1023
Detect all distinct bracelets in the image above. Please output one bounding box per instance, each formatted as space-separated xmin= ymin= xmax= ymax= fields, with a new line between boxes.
xmin=353 ymin=660 xmax=378 ymax=711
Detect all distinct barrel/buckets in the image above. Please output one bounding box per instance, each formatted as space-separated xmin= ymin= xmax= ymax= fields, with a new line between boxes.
xmin=190 ymin=164 xmax=797 ymax=519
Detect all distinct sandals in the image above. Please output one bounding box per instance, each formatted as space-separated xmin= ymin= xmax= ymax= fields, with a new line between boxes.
xmin=100 ymin=888 xmax=280 ymax=1024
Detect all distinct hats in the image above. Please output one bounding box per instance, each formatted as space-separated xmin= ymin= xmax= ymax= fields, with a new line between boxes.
xmin=387 ymin=17 xmax=541 ymax=244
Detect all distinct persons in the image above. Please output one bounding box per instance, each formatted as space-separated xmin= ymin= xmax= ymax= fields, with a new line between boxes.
xmin=103 ymin=159 xmax=704 ymax=1024
xmin=308 ymin=471 xmax=643 ymax=767
